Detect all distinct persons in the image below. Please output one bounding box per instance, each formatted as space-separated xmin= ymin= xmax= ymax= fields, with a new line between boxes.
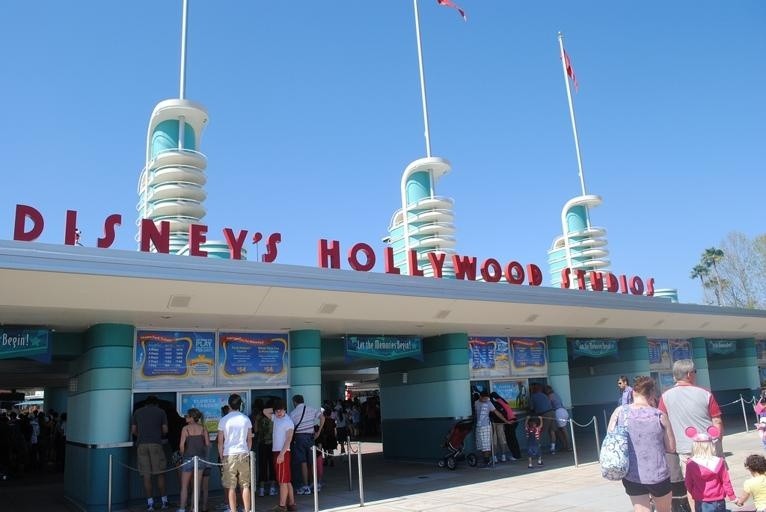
xmin=488 ymin=397 xmax=508 ymax=463
xmin=615 ymin=375 xmax=632 ymax=406
xmin=524 ymin=415 xmax=544 ymax=469
xmin=754 ymin=390 xmax=766 ymax=443
xmin=655 ymin=358 xmax=722 ymax=512
xmin=544 ymin=385 xmax=573 ymax=452
xmin=124 ymin=389 xmax=380 ymax=511
xmin=0 ymin=407 xmax=66 ymax=490
xmin=736 ymin=454 xmax=766 ymax=512
xmin=684 ymin=434 xmax=739 ymax=512
xmin=530 ymin=384 xmax=553 ymax=453
xmin=605 ymin=376 xmax=676 ymax=512
xmin=473 ymin=390 xmax=510 ymax=468
xmin=490 ymin=392 xmax=521 ymax=461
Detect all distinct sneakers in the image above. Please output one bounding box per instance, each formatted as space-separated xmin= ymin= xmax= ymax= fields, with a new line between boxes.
xmin=487 ymin=448 xmax=575 ymax=469
xmin=143 ymin=483 xmax=321 ymax=512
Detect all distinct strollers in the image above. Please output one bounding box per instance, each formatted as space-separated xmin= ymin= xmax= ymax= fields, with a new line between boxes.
xmin=434 ymin=417 xmax=481 ymax=471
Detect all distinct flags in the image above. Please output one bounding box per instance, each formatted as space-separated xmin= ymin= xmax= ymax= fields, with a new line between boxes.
xmin=433 ymin=0 xmax=466 ymax=20
xmin=560 ymin=47 xmax=577 ymax=92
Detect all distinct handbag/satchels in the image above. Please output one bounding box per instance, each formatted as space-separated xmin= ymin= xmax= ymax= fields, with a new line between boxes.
xmin=599 ymin=427 xmax=632 ymax=482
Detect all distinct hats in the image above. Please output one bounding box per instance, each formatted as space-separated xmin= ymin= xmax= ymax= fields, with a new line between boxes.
xmin=692 ymin=432 xmax=714 ymax=443
